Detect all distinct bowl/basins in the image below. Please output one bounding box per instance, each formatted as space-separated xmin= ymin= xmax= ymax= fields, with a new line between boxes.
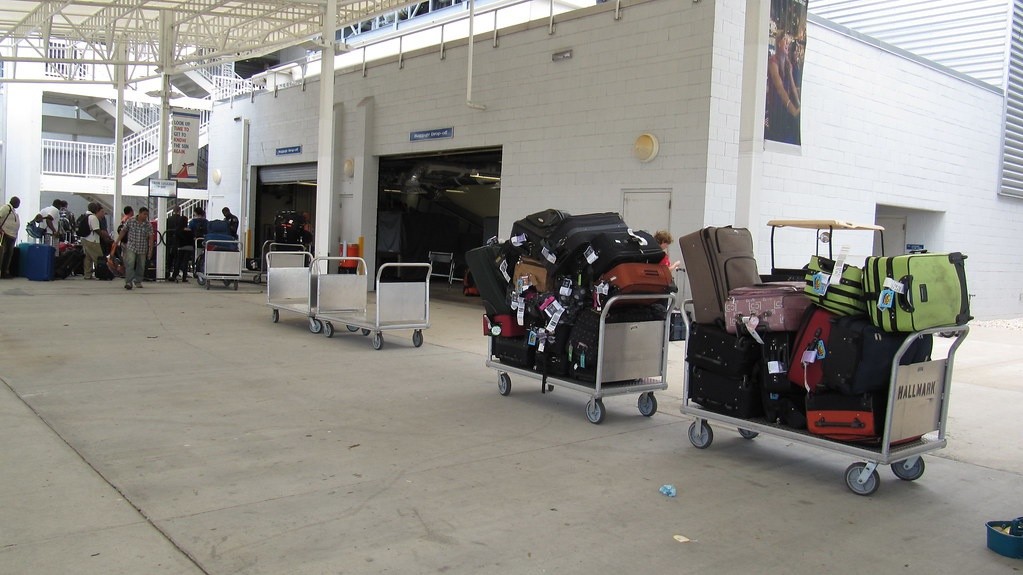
xmin=985 ymin=520 xmax=1023 ymax=559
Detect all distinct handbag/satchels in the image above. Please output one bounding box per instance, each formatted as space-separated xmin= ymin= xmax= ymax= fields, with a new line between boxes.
xmin=106 ymin=244 xmax=124 ymax=277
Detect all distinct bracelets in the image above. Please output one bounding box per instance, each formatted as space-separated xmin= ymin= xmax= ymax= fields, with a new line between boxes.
xmin=114 ymin=242 xmax=117 ymax=244
xmin=786 ymin=99 xmax=791 ymax=107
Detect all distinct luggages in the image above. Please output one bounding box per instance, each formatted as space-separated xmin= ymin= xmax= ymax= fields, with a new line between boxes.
xmin=28 ymin=244 xmax=56 ymax=281
xmin=195 ymin=219 xmax=237 ymax=275
xmin=96 ymin=256 xmax=114 ymax=281
xmin=467 ymin=210 xmax=675 ymax=386
xmin=9 ymin=246 xmax=20 ymax=277
xmin=18 ymin=242 xmax=31 ymax=277
xmin=681 ymin=224 xmax=976 ymax=445
xmin=55 ymin=246 xmax=84 ymax=278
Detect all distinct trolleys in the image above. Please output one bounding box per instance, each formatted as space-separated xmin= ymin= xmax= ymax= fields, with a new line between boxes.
xmin=314 ymin=255 xmax=433 ymax=352
xmin=253 ymin=239 xmax=305 ymax=285
xmin=427 ymin=250 xmax=464 ymax=285
xmin=264 ymin=250 xmax=360 ymax=334
xmin=483 ymin=292 xmax=680 ymax=424
xmin=194 ymin=237 xmax=245 ymax=291
xmin=679 ymin=296 xmax=972 ymax=499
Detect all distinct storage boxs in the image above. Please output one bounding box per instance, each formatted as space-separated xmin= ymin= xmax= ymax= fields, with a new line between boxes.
xmin=669 ymin=311 xmax=693 ymax=340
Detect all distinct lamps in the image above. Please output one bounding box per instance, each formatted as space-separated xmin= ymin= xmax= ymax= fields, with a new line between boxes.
xmin=343 ymin=158 xmax=354 ymax=178
xmin=213 ymin=170 xmax=222 ymax=184
xmin=634 ymin=133 xmax=660 ymax=162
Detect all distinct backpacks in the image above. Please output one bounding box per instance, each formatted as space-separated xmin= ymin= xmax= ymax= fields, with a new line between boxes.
xmin=75 ymin=212 xmax=93 ymax=237
xmin=117 ymin=222 xmax=129 ymax=243
xmin=26 ymin=217 xmax=53 ymax=238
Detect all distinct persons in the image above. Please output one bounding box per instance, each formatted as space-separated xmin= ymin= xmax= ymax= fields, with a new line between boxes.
xmin=121 ymin=205 xmax=134 ymax=268
xmin=0 ymin=196 xmax=20 ymax=278
xmin=222 ymin=207 xmax=239 ymax=248
xmin=165 ymin=207 xmax=196 ymax=282
xmin=80 ymin=203 xmax=114 ymax=280
xmin=188 ymin=208 xmax=210 ymax=279
xmin=36 ymin=199 xmax=63 ymax=256
xmin=767 ymin=33 xmax=806 ymax=146
xmin=111 ymin=207 xmax=153 ymax=290
xmin=58 ymin=200 xmax=75 ymax=244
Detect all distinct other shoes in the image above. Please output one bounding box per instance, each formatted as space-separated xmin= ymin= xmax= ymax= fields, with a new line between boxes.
xmin=124 ymin=284 xmax=133 ymax=290
xmin=136 ymin=283 xmax=143 ymax=288
xmin=169 ymin=277 xmax=176 ymax=280
xmin=182 ymin=278 xmax=188 ymax=282
